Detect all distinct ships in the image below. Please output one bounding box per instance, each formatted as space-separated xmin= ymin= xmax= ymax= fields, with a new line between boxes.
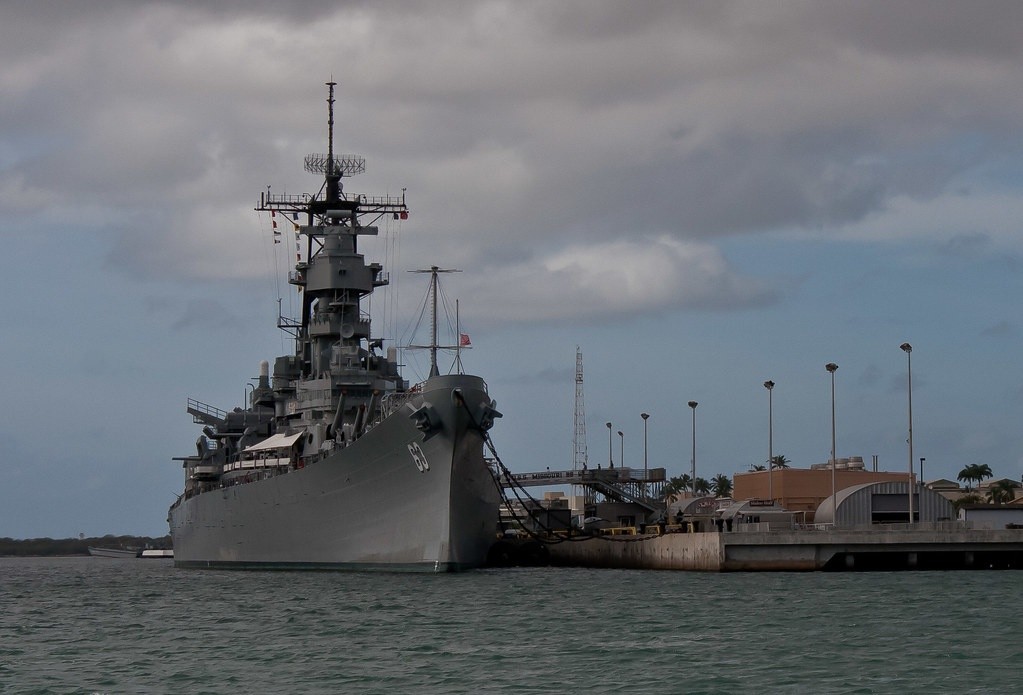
xmin=166 ymin=74 xmax=508 ymax=577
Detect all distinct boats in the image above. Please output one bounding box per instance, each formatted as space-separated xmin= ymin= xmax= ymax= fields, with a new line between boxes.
xmin=87 ymin=545 xmax=137 ymax=558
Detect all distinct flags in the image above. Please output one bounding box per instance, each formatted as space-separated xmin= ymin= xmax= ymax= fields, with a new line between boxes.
xmin=460 ymin=334 xmax=471 ymax=345
xmin=274 ymin=230 xmax=281 ymax=236
xmin=298 ymin=286 xmax=302 ymax=292
xmin=393 ymin=212 xmax=399 ymax=219
xmin=293 ymin=213 xmax=298 ymax=220
xmin=298 ymin=276 xmax=303 ymax=281
xmin=296 ymin=265 xmax=299 ymax=270
xmin=297 ymin=254 xmax=300 ymax=261
xmin=400 ymin=211 xmax=408 ymax=219
xmin=296 ymin=233 xmax=300 ymax=240
xmin=294 ymin=223 xmax=300 ymax=230
xmin=272 ymin=210 xmax=275 ymax=217
xmin=275 ymin=240 xmax=280 ymax=243
xmin=296 ymin=243 xmax=301 ymax=251
xmin=273 ymin=220 xmax=277 ymax=228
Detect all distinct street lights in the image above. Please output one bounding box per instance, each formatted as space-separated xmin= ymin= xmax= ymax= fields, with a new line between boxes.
xmin=900 ymin=341 xmax=914 ymax=523
xmin=687 ymin=400 xmax=699 ymax=497
xmin=617 ymin=431 xmax=624 ymax=467
xmin=825 ymin=361 xmax=840 ymax=529
xmin=605 ymin=422 xmax=614 ymax=469
xmin=764 ymin=380 xmax=775 ymax=500
xmin=920 ymin=457 xmax=925 ymax=486
xmin=640 ymin=412 xmax=650 ymax=481
